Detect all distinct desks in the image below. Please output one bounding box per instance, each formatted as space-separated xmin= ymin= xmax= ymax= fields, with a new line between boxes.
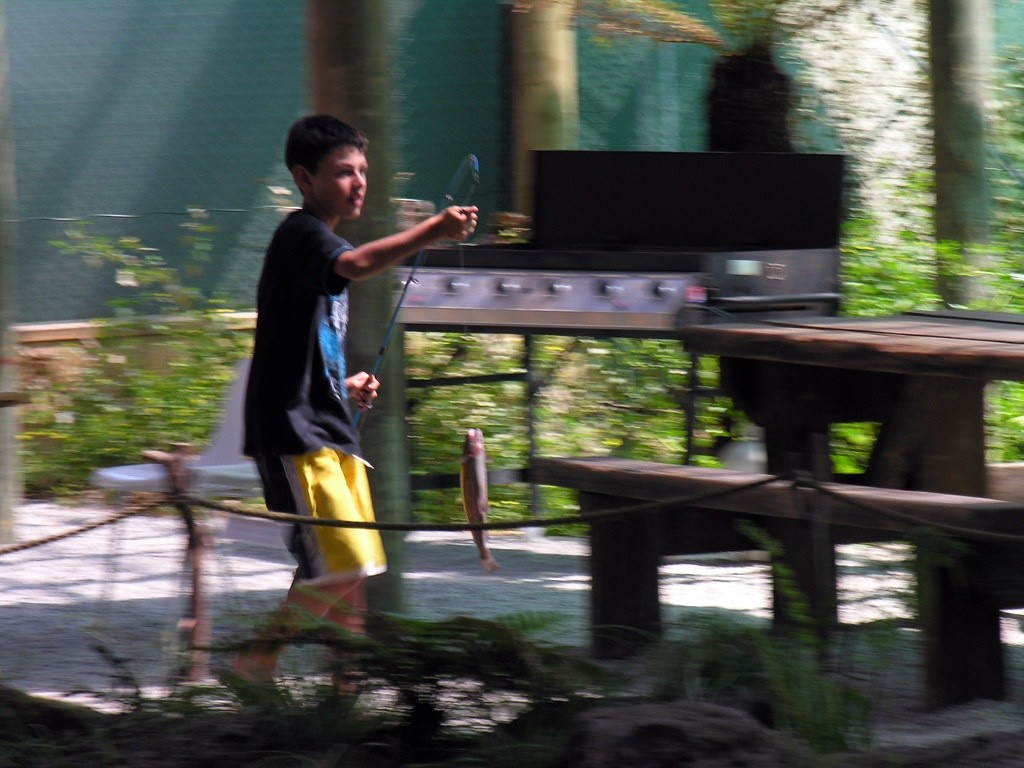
xmin=678 ymin=315 xmax=1024 ymax=634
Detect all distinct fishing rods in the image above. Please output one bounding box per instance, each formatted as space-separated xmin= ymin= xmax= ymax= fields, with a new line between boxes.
xmin=351 ymin=151 xmax=480 ymax=433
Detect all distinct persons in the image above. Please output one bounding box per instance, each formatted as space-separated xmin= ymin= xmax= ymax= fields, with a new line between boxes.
xmin=236 ymin=112 xmax=477 ymax=688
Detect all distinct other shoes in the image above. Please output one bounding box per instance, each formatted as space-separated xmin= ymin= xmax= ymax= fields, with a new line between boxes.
xmin=333 ymin=664 xmax=371 ymax=696
xmin=210 ymin=661 xmax=287 ymax=713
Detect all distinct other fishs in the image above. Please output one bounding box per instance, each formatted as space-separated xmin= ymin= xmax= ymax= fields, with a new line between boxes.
xmin=460 ymin=427 xmax=504 ymax=575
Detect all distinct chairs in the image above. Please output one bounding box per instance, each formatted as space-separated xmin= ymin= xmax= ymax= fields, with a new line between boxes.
xmin=91 ymin=359 xmax=249 ymax=632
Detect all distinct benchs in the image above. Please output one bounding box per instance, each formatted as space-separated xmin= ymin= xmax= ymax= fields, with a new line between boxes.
xmin=511 ymin=454 xmax=1023 ymax=705
